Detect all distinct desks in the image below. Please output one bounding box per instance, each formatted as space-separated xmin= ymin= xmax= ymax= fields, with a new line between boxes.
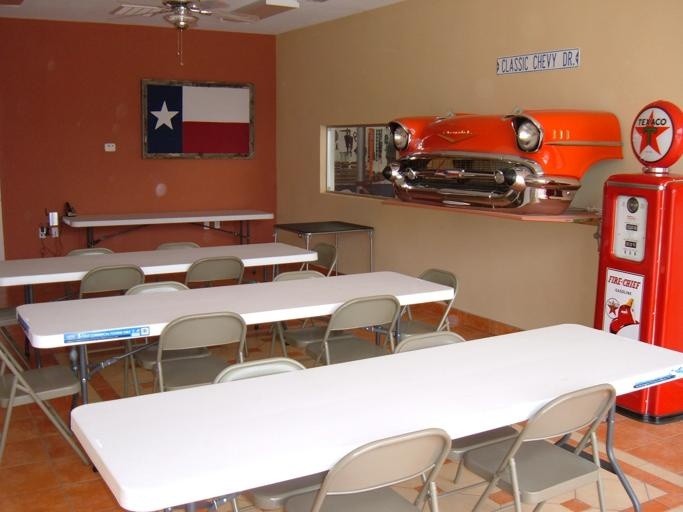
xmin=70 ymin=323 xmax=683 ymax=510
xmin=61 ymin=208 xmax=274 ymax=250
xmin=272 ymin=218 xmax=375 ymax=280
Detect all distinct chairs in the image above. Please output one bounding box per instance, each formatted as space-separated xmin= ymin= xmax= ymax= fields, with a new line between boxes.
xmin=212 ymin=358 xmax=329 ymax=511
xmin=394 ymin=333 xmax=520 ymax=512
xmin=463 ymin=382 xmax=615 ymax=511
xmin=4 ymin=242 xmax=457 ymax=470
xmin=283 ymin=429 xmax=453 ymax=512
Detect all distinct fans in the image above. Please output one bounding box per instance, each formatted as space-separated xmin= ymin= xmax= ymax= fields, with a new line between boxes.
xmin=106 ymin=0 xmax=263 ymax=33
